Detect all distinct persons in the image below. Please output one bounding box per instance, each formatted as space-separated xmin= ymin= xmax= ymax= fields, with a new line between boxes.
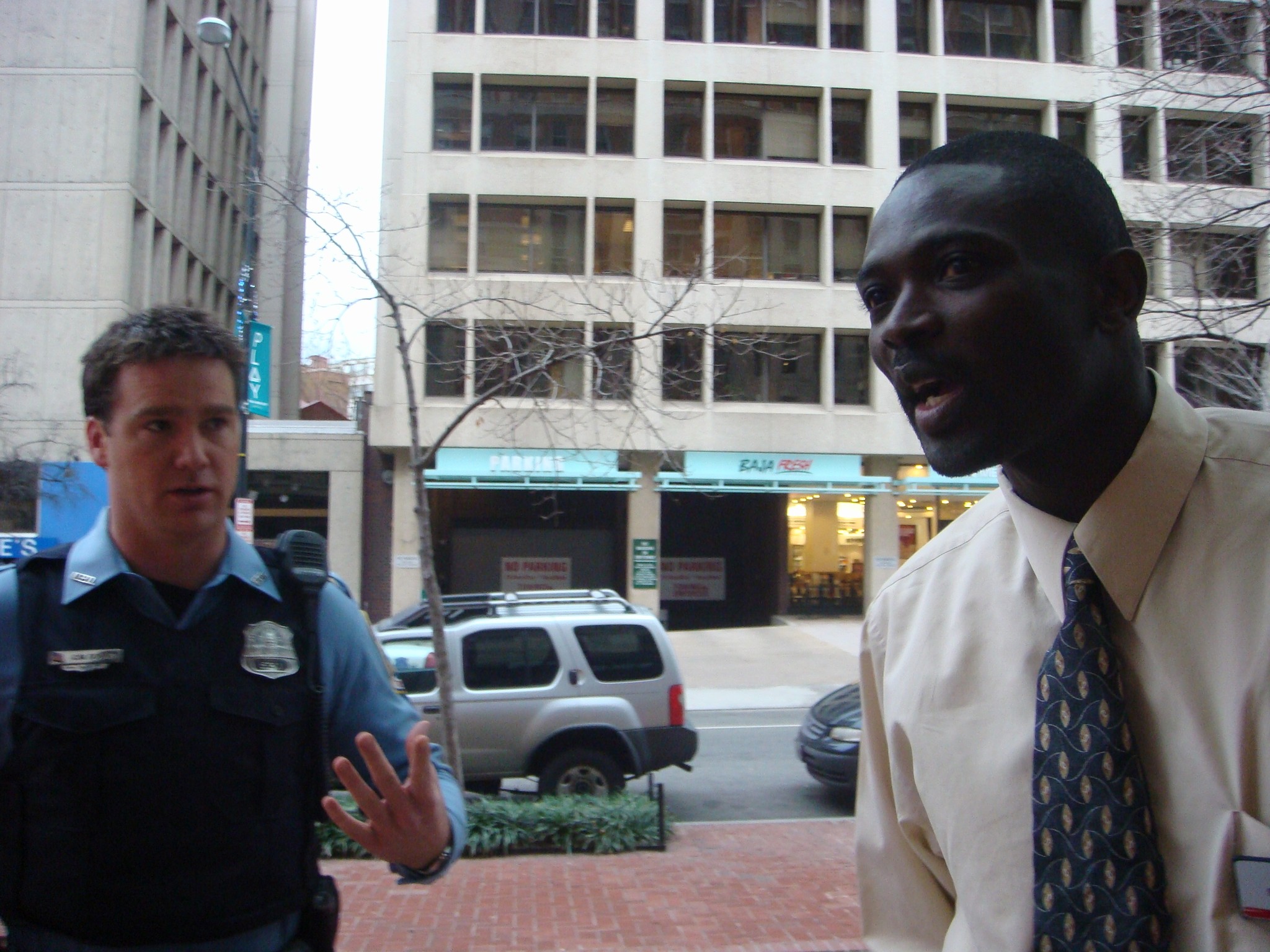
xmin=857 ymin=130 xmax=1269 ymax=952
xmin=0 ymin=306 xmax=468 ymax=949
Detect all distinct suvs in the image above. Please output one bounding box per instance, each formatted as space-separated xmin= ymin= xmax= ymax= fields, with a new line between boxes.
xmin=366 ymin=588 xmax=698 ymax=798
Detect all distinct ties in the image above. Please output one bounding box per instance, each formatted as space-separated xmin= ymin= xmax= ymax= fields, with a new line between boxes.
xmin=1033 ymin=533 xmax=1170 ymax=952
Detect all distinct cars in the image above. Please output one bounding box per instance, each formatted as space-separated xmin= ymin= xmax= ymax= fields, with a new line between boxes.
xmin=792 ymin=679 xmax=863 ymax=800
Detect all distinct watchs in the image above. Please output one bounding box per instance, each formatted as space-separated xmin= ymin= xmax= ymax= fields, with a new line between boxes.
xmin=390 ymin=846 xmax=453 ymax=879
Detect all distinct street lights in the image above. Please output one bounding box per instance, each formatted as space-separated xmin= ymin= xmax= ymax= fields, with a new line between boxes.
xmin=192 ymin=13 xmax=267 ymax=543
